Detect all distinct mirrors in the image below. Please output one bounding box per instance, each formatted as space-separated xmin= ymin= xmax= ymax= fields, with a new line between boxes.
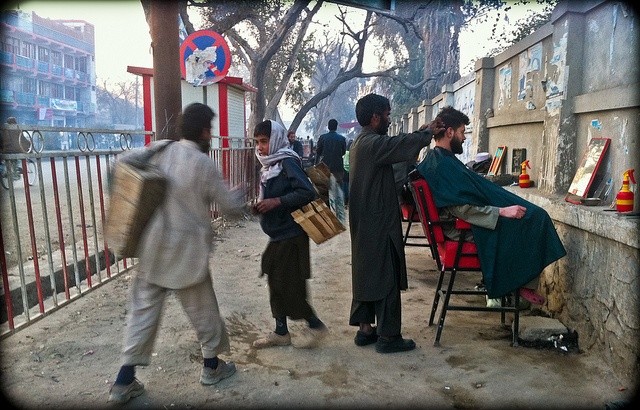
xmin=564 ymin=138 xmax=611 ymax=204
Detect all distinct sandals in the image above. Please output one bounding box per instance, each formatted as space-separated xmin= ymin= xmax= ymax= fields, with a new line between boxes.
xmin=518 ymin=287 xmax=544 ymax=306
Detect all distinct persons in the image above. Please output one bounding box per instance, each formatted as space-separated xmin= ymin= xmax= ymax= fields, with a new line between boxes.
xmin=286 ymin=131 xmax=304 ymax=159
xmin=95 ymin=102 xmax=239 ymax=406
xmin=415 ymin=107 xmax=568 ymax=307
xmin=250 ymin=119 xmax=331 ymax=354
xmin=312 ymin=118 xmax=347 ymax=227
xmin=7 ymin=116 xmax=17 ymax=124
xmin=348 ymin=92 xmax=448 ymax=355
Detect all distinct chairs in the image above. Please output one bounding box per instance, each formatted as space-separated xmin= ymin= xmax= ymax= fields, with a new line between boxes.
xmin=400 ymin=194 xmax=434 ymax=260
xmin=412 ymin=178 xmax=520 ymax=347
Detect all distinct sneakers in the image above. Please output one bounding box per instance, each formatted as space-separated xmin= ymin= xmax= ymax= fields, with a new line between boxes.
xmin=109 ymin=378 xmax=144 ymax=404
xmin=376 ymin=335 xmax=417 ymax=353
xmin=253 ymin=332 xmax=290 ymax=348
xmin=355 ymin=328 xmax=378 ymax=346
xmin=294 ymin=326 xmax=329 ymax=344
xmin=200 ymin=359 xmax=236 ymax=385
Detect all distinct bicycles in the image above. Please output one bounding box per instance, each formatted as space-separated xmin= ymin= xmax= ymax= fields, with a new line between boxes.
xmin=0 ymin=149 xmax=36 ymax=189
